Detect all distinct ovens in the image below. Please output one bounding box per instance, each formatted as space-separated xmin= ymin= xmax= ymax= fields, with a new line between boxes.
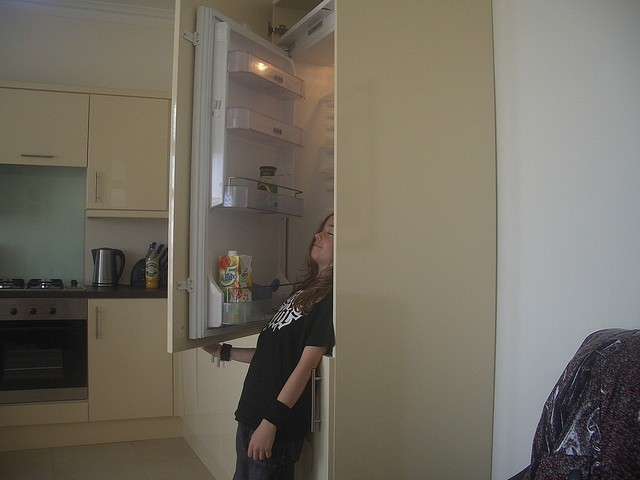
xmin=0 ymin=295 xmax=89 ymax=404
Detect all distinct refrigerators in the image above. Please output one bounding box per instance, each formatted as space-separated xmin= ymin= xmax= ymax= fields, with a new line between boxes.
xmin=168 ymin=0 xmax=336 ymax=480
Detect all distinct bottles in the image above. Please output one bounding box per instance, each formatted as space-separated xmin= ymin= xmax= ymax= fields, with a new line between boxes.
xmin=217 ymin=250 xmax=253 ymax=303
xmin=145 ymin=244 xmax=160 ymax=288
xmin=257 ymin=166 xmax=278 ymax=214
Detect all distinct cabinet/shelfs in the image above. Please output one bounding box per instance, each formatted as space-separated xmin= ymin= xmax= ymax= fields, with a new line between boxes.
xmin=1 ymin=88 xmax=89 ymax=169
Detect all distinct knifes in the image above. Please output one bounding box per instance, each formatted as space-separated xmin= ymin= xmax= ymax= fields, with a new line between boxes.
xmin=150 ymin=242 xmax=168 ymax=266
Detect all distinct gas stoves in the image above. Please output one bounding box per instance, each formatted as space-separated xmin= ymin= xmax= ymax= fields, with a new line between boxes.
xmin=0 ymin=278 xmax=85 ymax=293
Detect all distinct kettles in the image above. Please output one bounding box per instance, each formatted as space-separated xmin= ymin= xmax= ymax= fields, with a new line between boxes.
xmin=91 ymin=247 xmax=125 ymax=286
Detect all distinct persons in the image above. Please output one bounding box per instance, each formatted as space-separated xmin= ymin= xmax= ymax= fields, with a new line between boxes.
xmin=197 ymin=212 xmax=334 ymax=480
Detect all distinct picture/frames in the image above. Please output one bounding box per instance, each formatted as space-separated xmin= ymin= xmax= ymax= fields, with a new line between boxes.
xmin=87 ymin=300 xmax=173 ymax=422
xmin=86 ymin=93 xmax=168 ymax=214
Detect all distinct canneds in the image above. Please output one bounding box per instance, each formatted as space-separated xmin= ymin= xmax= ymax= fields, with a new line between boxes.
xmin=256 ymin=165 xmax=279 ymax=195
